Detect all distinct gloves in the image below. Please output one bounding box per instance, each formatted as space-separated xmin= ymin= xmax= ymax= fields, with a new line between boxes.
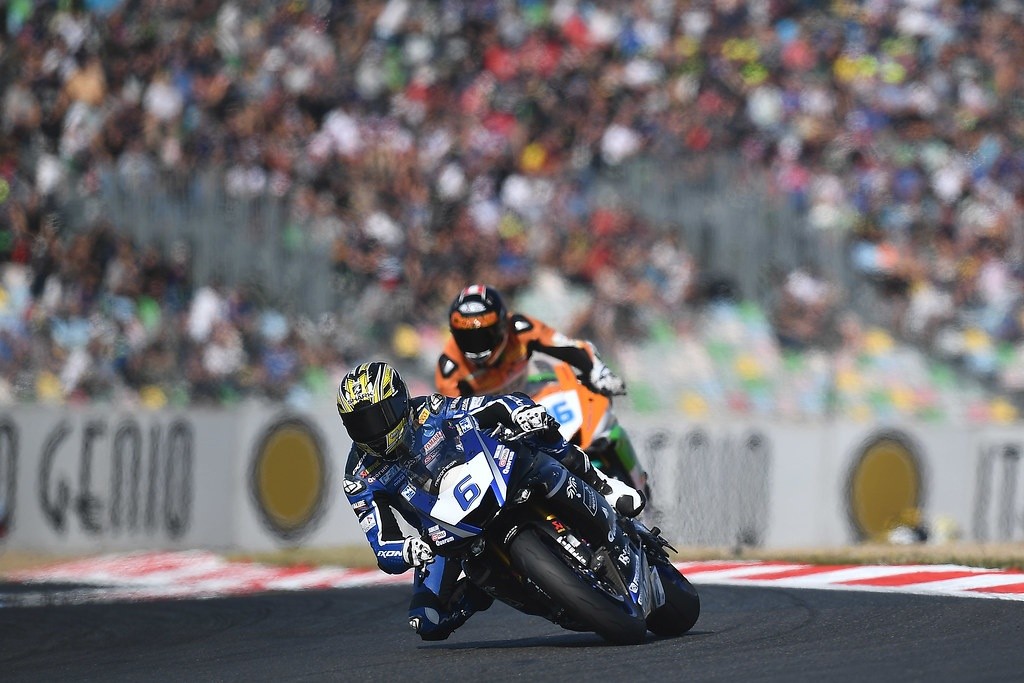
xmin=403 ymin=535 xmax=436 ymax=567
xmin=512 ymin=404 xmax=547 ymax=438
xmin=586 ymin=367 xmax=628 ymax=398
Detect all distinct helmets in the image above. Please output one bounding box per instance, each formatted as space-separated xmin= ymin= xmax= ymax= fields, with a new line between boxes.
xmin=449 ymin=284 xmax=509 ymax=367
xmin=336 ymin=361 xmax=414 ymax=459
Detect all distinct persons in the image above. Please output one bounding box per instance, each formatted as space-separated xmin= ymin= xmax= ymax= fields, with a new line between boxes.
xmin=338 ymin=363 xmax=646 ymax=640
xmin=436 ymin=282 xmax=654 ymax=511
xmin=0 ymin=0 xmax=1024 ymax=417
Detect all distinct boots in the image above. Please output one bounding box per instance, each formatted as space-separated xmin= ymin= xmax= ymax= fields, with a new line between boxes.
xmin=560 ymin=444 xmax=646 ymax=518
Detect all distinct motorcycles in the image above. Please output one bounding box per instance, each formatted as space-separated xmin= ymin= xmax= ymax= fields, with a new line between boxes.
xmin=494 ymin=356 xmax=635 ymax=501
xmin=399 ymin=412 xmax=702 ymax=647
